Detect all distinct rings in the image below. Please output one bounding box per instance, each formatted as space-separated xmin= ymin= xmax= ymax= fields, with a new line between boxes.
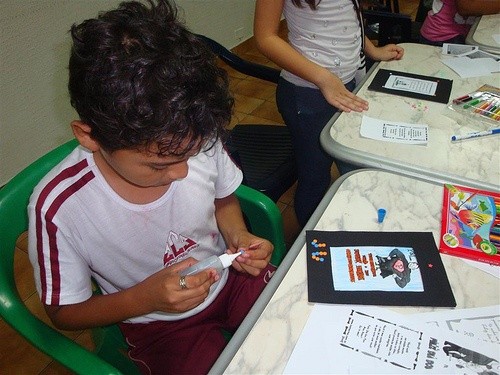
xmin=179 ymin=276 xmax=186 ymax=290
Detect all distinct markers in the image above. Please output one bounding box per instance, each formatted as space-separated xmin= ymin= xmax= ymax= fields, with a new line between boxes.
xmin=453 ymin=92 xmax=500 ymax=121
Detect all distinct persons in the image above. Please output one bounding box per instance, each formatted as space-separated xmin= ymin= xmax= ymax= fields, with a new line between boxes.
xmin=26 ymin=0 xmax=277 ymax=375
xmin=420 ymin=0 xmax=500 ymax=47
xmin=254 ymin=0 xmax=405 ymax=229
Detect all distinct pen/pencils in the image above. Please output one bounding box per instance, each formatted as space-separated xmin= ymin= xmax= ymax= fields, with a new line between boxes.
xmin=452 ymin=127 xmax=500 ymax=147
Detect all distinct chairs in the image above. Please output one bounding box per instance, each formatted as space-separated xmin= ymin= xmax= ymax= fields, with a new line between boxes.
xmin=363 ymin=1 xmax=422 ymax=47
xmin=191 ymin=33 xmax=298 ymax=204
xmin=0 ymin=137 xmax=285 ymax=375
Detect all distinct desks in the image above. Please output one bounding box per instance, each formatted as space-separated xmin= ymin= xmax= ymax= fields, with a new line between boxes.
xmin=205 ymin=167 xmax=500 ymax=375
xmin=464 ymin=13 xmax=500 ymax=56
xmin=319 ymin=42 xmax=500 ymax=192
